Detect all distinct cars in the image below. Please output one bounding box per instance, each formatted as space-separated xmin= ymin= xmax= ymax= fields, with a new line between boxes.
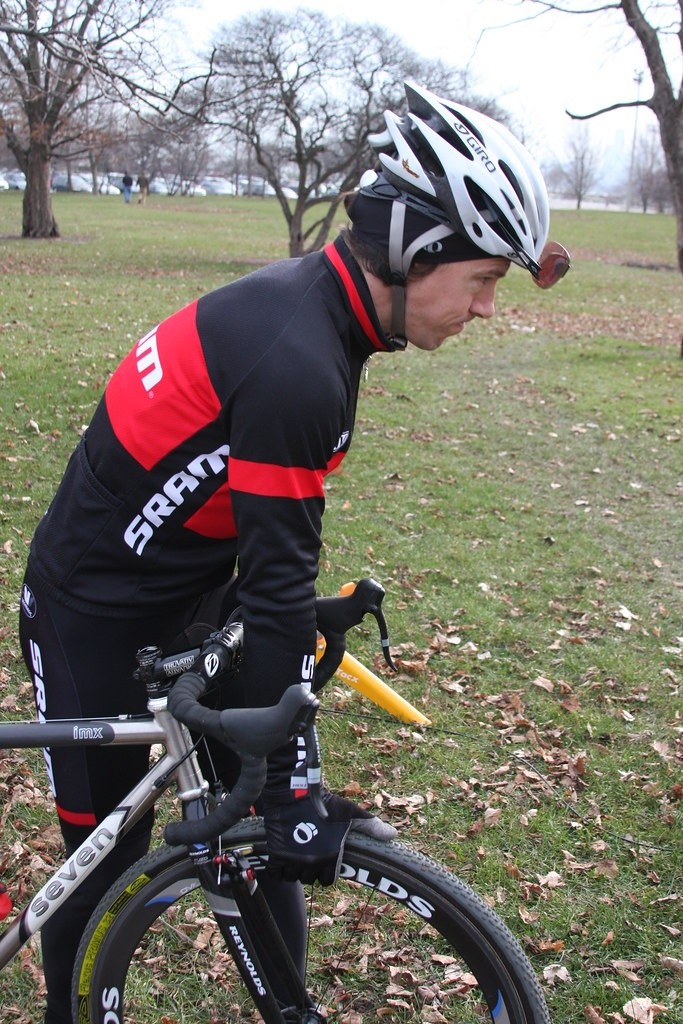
xmin=0 ymin=173 xmax=339 ymax=198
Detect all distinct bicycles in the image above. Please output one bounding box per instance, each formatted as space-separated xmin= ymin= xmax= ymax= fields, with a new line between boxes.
xmin=1 ymin=578 xmax=551 ymax=1024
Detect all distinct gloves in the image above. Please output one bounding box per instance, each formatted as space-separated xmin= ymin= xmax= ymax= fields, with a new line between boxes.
xmin=266 ymin=787 xmax=401 ymax=886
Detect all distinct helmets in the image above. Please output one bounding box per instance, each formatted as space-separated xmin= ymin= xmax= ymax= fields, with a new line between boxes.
xmin=342 ymin=80 xmax=549 ymax=280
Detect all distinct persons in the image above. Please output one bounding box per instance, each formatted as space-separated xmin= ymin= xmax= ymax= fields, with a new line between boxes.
xmin=123 ymin=171 xmax=134 ymax=202
xmin=136 ymin=173 xmax=150 ymax=203
xmin=19 ymin=80 xmax=572 ymax=1024
